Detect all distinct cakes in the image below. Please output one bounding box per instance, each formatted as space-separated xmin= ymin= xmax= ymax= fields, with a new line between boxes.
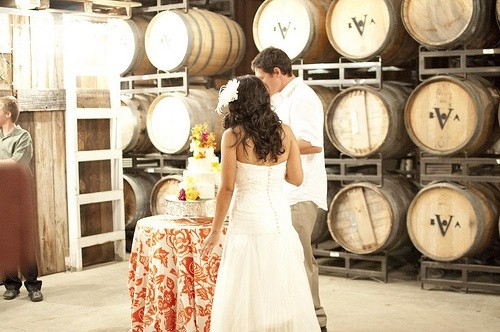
xmin=182 ymin=123 xmax=221 ymax=186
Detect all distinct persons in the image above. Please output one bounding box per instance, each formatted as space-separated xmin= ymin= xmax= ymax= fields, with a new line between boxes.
xmin=0 ymin=96 xmax=42 ymax=301
xmin=199 ymin=73 xmax=321 ymax=332
xmin=250 ymin=47 xmax=329 ymax=332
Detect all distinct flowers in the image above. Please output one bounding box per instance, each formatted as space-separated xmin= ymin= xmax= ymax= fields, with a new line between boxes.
xmin=176 ymin=121 xmax=223 ymax=203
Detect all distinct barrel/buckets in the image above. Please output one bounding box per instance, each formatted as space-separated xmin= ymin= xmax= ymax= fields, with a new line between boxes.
xmin=121 ymin=93 xmax=156 ymax=153
xmin=252 ymin=0 xmax=341 ymax=64
xmin=144 ymin=6 xmax=247 ymax=77
xmin=307 ymin=180 xmax=343 ymax=244
xmin=309 ymin=86 xmax=338 ymax=157
xmin=404 ymin=73 xmax=499 ymax=157
xmin=400 ymin=0 xmax=498 ymax=49
xmin=325 ymin=0 xmax=425 ymax=67
xmin=406 ymin=182 xmax=500 ymax=262
xmin=112 ymin=15 xmax=158 ymax=76
xmin=326 ymin=82 xmax=414 ymax=156
xmin=150 ymin=174 xmax=185 ymax=215
xmin=122 ymin=172 xmax=160 ymax=231
xmin=146 ymin=89 xmax=229 ymax=155
xmin=327 ymin=177 xmax=418 ymax=254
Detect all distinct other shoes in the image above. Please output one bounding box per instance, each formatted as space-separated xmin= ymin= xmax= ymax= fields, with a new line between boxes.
xmin=30 ymin=290 xmax=43 ymax=302
xmin=4 ymin=289 xmax=20 ymax=299
xmin=321 ymin=326 xmax=327 ymax=332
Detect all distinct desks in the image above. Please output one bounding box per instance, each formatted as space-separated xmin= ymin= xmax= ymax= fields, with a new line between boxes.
xmin=127 ymin=215 xmax=227 ymax=332
